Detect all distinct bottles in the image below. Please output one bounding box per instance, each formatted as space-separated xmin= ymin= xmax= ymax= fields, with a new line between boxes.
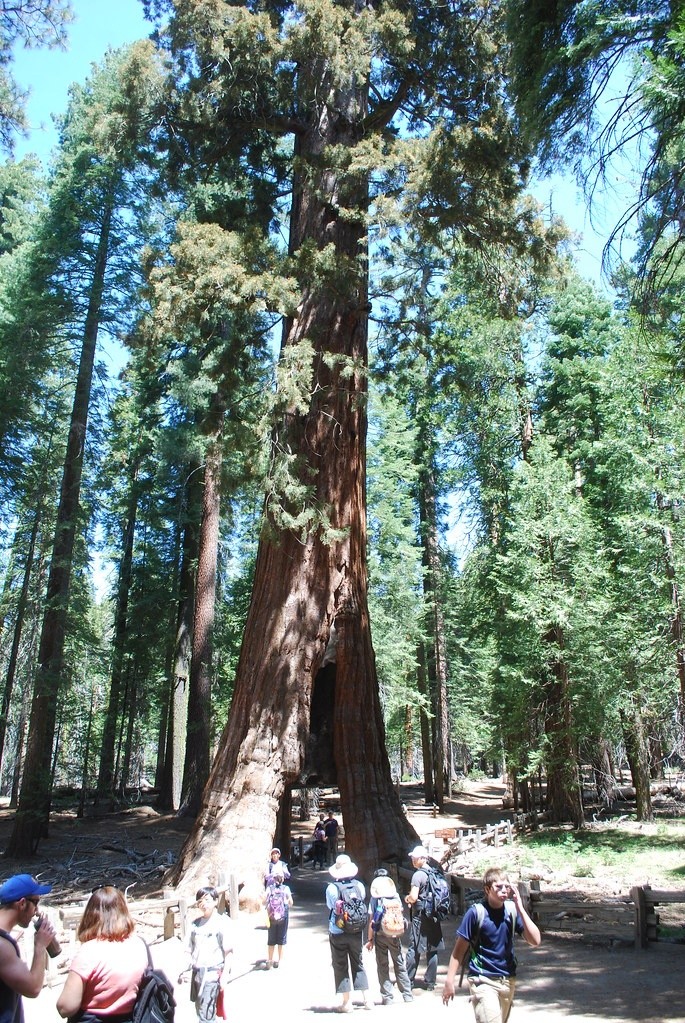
xmin=336 ymin=900 xmax=345 ymax=914
xmin=371 ymin=906 xmax=385 ymax=931
xmin=425 ymin=891 xmax=433 ymax=913
xmin=32 ymin=914 xmax=62 ymax=958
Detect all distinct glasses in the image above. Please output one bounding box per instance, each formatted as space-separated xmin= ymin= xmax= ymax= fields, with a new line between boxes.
xmin=495 ymin=884 xmax=510 ymax=889
xmin=91 ymin=884 xmax=118 ymax=893
xmin=22 ymin=896 xmax=40 ymax=907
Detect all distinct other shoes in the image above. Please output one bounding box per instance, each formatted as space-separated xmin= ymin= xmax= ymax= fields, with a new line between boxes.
xmin=364 ymin=1001 xmax=375 ymax=1011
xmin=382 ymin=998 xmax=392 ymax=1005
xmin=402 ymin=994 xmax=413 ymax=1002
xmin=273 ymin=961 xmax=279 ymax=968
xmin=425 ymin=985 xmax=433 ymax=990
xmin=337 ymin=1001 xmax=354 ymax=1013
xmin=265 ymin=961 xmax=272 ymax=970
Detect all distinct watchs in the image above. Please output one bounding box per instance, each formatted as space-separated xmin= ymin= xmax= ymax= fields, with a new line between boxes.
xmin=368 ymin=939 xmax=372 ymax=941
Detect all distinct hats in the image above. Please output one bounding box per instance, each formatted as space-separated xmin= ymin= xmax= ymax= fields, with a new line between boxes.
xmin=328 ymin=854 xmax=358 ymax=878
xmin=270 ymin=848 xmax=281 ymax=855
xmin=408 ymin=846 xmax=429 ymax=860
xmin=370 ymin=876 xmax=397 ymax=897
xmin=273 ymin=871 xmax=284 ymax=878
xmin=1 ymin=874 xmax=52 ymax=904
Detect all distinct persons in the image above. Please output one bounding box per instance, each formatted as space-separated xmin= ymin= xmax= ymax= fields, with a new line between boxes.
xmin=188 ymin=885 xmax=233 ymax=1023
xmin=404 ymin=846 xmax=438 ymax=990
xmin=312 ymin=811 xmax=339 ymax=869
xmin=365 ymin=868 xmax=414 ymax=1005
xmin=441 ymin=869 xmax=541 ymax=1023
xmin=0 ymin=873 xmax=59 ymax=1023
xmin=56 ymin=886 xmax=152 ymax=1023
xmin=262 ymin=871 xmax=294 ymax=965
xmin=325 ymin=854 xmax=374 ymax=1013
xmin=264 ymin=847 xmax=291 ymax=881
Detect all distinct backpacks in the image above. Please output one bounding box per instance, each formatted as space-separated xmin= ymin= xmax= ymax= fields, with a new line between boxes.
xmin=132 ymin=935 xmax=178 ymax=1023
xmin=417 ymin=867 xmax=452 ymax=921
xmin=265 ymin=885 xmax=287 ymax=923
xmin=373 ymin=898 xmax=405 ymax=937
xmin=328 ymin=880 xmax=368 ymax=933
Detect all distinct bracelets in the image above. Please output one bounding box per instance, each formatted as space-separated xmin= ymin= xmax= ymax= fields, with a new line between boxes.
xmin=406 ymin=898 xmax=409 ymax=901
